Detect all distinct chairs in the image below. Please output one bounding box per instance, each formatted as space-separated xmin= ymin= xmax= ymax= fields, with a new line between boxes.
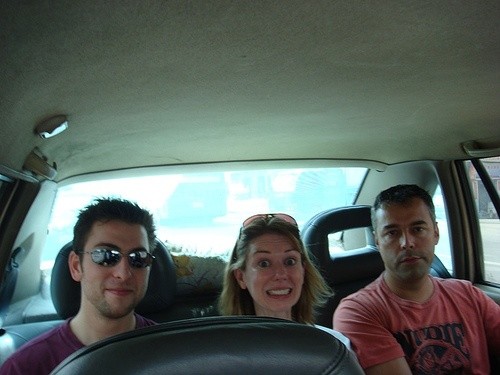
xmin=48 ymin=314 xmax=365 ymax=375
xmin=0 ymin=236 xmax=196 ymax=366
xmin=299 ymin=204 xmax=454 ymax=331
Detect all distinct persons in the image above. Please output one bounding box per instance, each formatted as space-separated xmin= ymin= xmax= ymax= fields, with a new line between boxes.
xmin=0 ymin=197 xmax=166 ymax=374
xmin=332 ymin=183 xmax=500 ymax=375
xmin=214 ymin=213 xmax=367 ymax=375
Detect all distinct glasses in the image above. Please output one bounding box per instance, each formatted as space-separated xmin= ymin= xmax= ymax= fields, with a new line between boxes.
xmin=244 ymin=213 xmax=299 ymax=231
xmin=77 ymin=247 xmax=155 ymax=268
